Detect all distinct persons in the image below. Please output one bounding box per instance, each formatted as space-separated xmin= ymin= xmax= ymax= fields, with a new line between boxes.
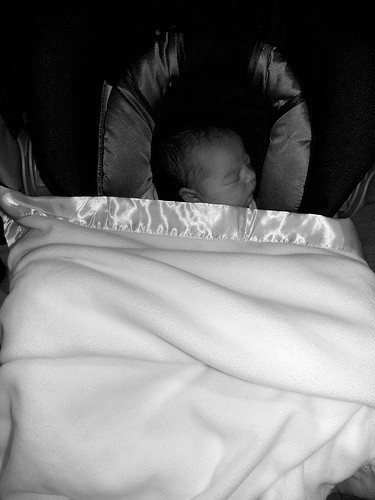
xmin=165 ymin=125 xmax=258 ymax=209
